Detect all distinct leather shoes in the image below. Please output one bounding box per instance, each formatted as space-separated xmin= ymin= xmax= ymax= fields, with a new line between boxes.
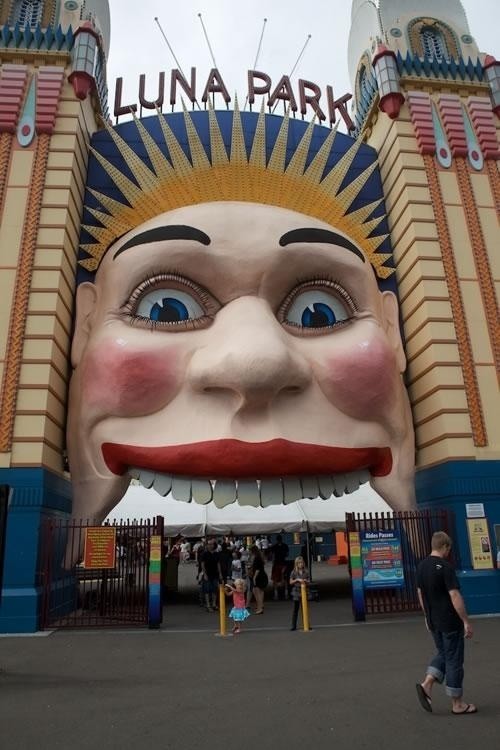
xmin=253 ymin=607 xmax=264 ymax=612
xmin=253 ymin=609 xmax=263 ymax=615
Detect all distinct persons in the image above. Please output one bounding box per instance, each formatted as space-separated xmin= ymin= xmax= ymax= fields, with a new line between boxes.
xmin=95 ymin=534 xmax=318 ymax=636
xmin=415 ymin=529 xmax=479 ymax=717
xmin=63 ymin=93 xmax=427 ymax=568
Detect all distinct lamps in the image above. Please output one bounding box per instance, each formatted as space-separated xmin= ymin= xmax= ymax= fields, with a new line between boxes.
xmin=68 ymin=21 xmax=98 ymax=99
xmin=372 ymin=41 xmax=405 ymax=118
xmin=485 ymin=54 xmax=500 ymax=119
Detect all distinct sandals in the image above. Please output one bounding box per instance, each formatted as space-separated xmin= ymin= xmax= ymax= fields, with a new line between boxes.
xmin=206 ymin=606 xmax=214 ymax=613
xmin=212 ymin=604 xmax=219 ymax=611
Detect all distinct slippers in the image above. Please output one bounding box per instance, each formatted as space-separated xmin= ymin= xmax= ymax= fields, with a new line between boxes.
xmin=416 ymin=684 xmax=434 ymax=713
xmin=451 ymin=703 xmax=478 ymax=715
